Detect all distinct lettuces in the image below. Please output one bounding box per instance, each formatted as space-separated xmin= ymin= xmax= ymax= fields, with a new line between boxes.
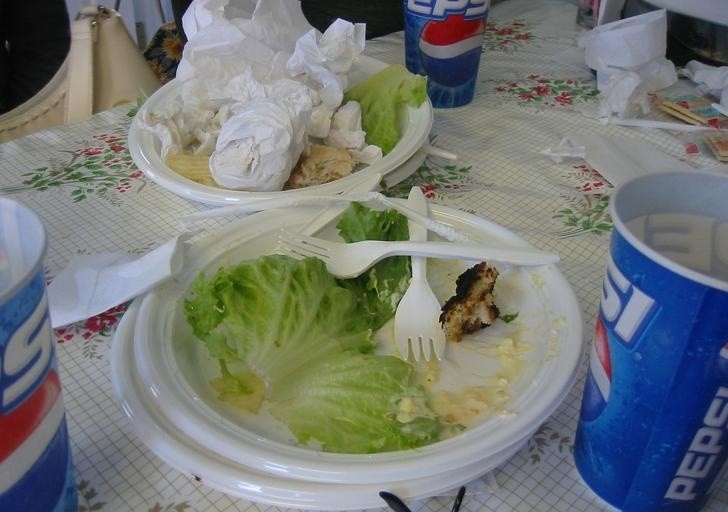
xmin=341 ymin=61 xmax=434 ymax=152
xmin=188 ymin=256 xmax=439 ymax=452
xmin=338 ymin=197 xmax=416 ymax=335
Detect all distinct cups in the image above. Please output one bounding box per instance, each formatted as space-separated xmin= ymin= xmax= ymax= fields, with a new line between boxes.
xmin=1 ymin=194 xmax=79 ymax=512
xmin=403 ymin=0 xmax=491 ymax=109
xmin=570 ymin=169 xmax=726 ymax=512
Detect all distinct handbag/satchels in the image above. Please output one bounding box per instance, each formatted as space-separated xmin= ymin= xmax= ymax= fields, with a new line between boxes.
xmin=1 ymin=2 xmax=162 ymax=145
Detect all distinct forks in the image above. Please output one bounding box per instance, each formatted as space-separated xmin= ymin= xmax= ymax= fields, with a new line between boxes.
xmin=274 ymin=184 xmax=562 ymax=366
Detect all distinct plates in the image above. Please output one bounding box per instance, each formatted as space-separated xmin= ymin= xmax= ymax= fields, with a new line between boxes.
xmin=108 ymin=201 xmax=584 ymax=511
xmin=129 ymin=51 xmax=432 ymax=203
